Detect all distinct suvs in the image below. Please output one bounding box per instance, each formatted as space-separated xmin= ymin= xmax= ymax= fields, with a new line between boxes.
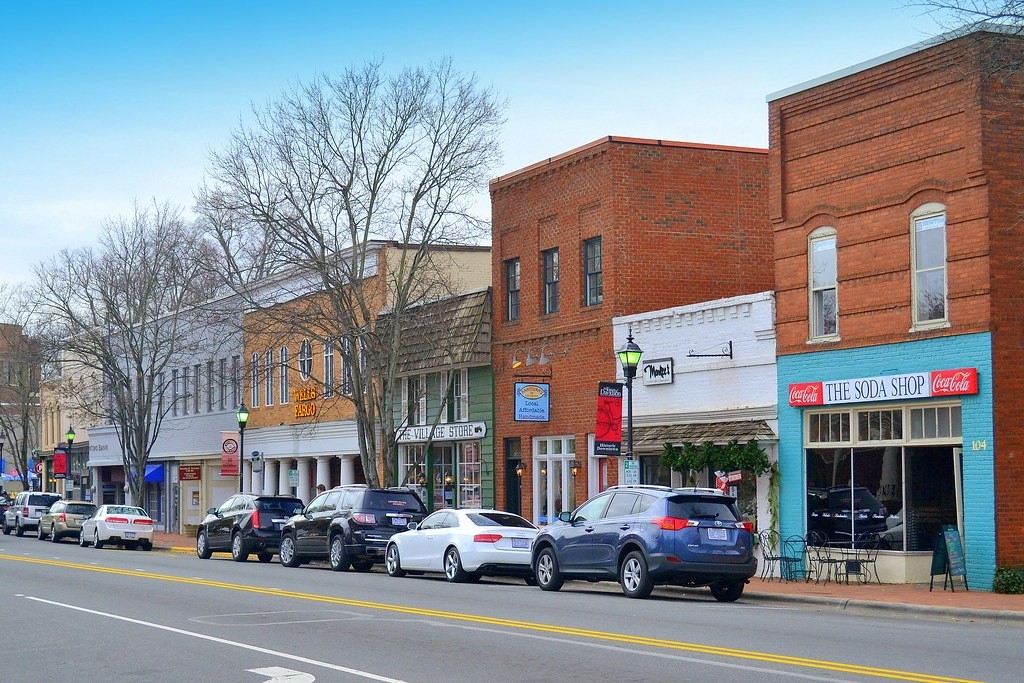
xmin=278 ymin=484 xmax=429 ymax=572
xmin=195 ymin=491 xmax=306 ymax=562
xmin=530 ymin=483 xmax=758 ymax=603
xmin=807 ymin=483 xmax=888 ymax=545
xmin=1 ymin=491 xmax=63 ymax=538
xmin=0 ymin=489 xmax=14 ymax=525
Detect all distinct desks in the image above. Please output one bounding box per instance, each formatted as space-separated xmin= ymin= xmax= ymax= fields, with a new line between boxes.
xmin=781 ymin=539 xmax=808 ymax=584
xmin=826 ymin=541 xmax=869 ymax=586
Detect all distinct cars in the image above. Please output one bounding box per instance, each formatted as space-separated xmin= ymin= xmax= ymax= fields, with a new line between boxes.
xmin=383 ymin=507 xmax=541 ymax=587
xmin=36 ymin=499 xmax=99 ymax=543
xmin=78 ymin=504 xmax=155 ymax=551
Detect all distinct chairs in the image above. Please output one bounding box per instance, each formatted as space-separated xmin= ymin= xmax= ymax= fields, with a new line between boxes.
xmin=759 ymin=529 xmax=881 ymax=586
xmin=809 ymin=530 xmax=894 ymax=549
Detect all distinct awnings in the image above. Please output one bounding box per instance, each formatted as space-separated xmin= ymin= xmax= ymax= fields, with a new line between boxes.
xmin=127 ymin=463 xmax=164 ymax=483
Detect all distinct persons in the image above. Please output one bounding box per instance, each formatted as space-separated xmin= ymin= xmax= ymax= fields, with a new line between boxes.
xmin=315 ymin=484 xmax=326 ymax=494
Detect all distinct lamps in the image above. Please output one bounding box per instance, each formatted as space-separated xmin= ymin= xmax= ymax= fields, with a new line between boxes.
xmin=538 ymin=345 xmax=568 ymax=365
xmin=444 ymin=470 xmax=455 ymax=488
xmin=525 ymin=345 xmax=553 ymax=366
xmin=515 ymin=460 xmax=527 ymax=478
xmin=417 ymin=471 xmax=428 ymax=491
xmin=512 ymin=349 xmax=538 ymax=369
xmin=569 ymin=459 xmax=581 ymax=477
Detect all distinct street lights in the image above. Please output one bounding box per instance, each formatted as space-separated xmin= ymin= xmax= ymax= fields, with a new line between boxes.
xmin=0 ymin=429 xmax=6 ymax=490
xmin=234 ymin=398 xmax=251 ymax=494
xmin=615 ymin=326 xmax=644 ymax=486
xmin=65 ymin=422 xmax=76 ymax=500
